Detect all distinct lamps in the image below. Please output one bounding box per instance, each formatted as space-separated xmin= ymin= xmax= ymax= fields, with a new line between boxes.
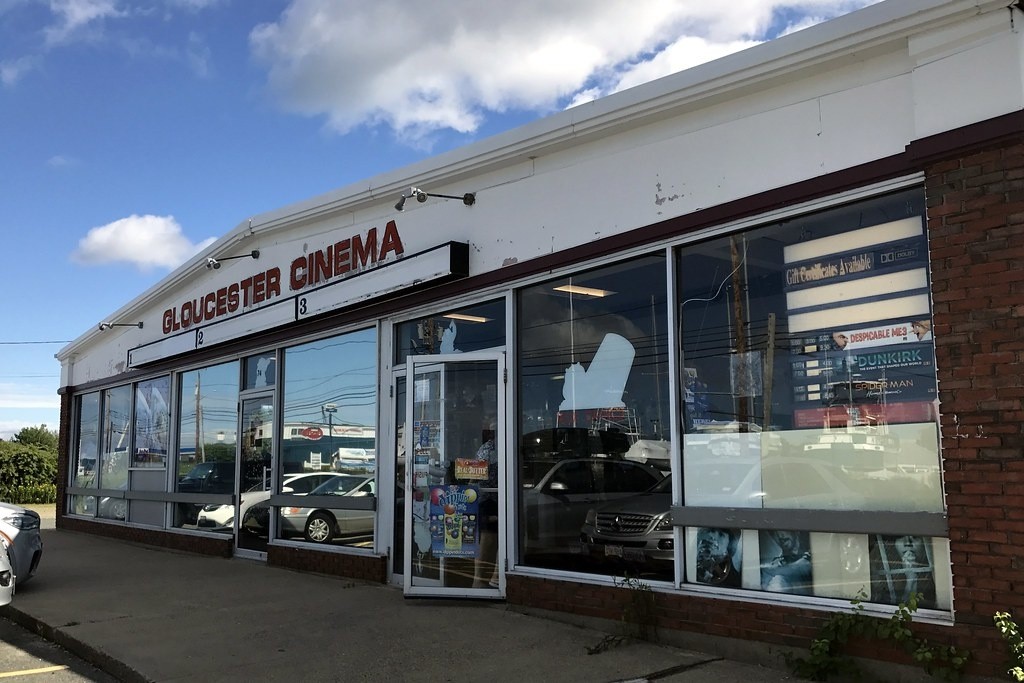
xmin=395 ymin=187 xmax=475 ymax=211
xmin=99 ymin=321 xmax=143 ymax=331
xmin=206 ymin=251 xmax=260 ymax=271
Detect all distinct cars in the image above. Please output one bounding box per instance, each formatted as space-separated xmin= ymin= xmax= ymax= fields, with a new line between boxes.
xmin=0 ymin=499 xmax=44 ymax=620
xmin=75 ymin=427 xmax=846 ymax=582
